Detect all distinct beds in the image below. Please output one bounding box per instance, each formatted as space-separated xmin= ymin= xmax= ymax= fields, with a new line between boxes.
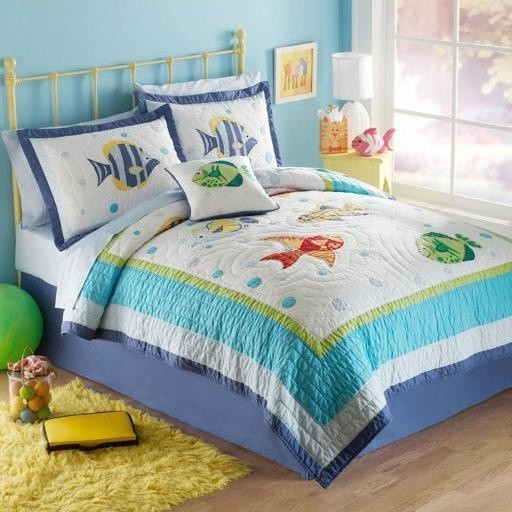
xmin=0 ymin=27 xmax=512 ymax=481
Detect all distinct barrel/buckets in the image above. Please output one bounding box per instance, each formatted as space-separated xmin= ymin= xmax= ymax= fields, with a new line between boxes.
xmin=7 ymin=370 xmax=55 ymax=424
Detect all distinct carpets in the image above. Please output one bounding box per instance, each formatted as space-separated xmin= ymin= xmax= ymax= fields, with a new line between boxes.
xmin=1 ymin=376 xmax=257 ymax=512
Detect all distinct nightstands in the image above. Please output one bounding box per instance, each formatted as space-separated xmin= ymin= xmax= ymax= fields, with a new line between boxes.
xmin=320 ymin=149 xmax=393 ymax=194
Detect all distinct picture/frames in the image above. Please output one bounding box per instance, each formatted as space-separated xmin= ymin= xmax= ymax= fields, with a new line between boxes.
xmin=273 ymin=41 xmax=318 ymax=105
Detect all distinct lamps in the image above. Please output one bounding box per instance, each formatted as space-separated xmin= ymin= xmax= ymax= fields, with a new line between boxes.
xmin=331 ymin=51 xmax=375 ymax=147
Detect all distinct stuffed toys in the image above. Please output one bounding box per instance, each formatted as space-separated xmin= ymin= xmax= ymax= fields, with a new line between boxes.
xmin=351 ymin=127 xmax=397 ymax=156
